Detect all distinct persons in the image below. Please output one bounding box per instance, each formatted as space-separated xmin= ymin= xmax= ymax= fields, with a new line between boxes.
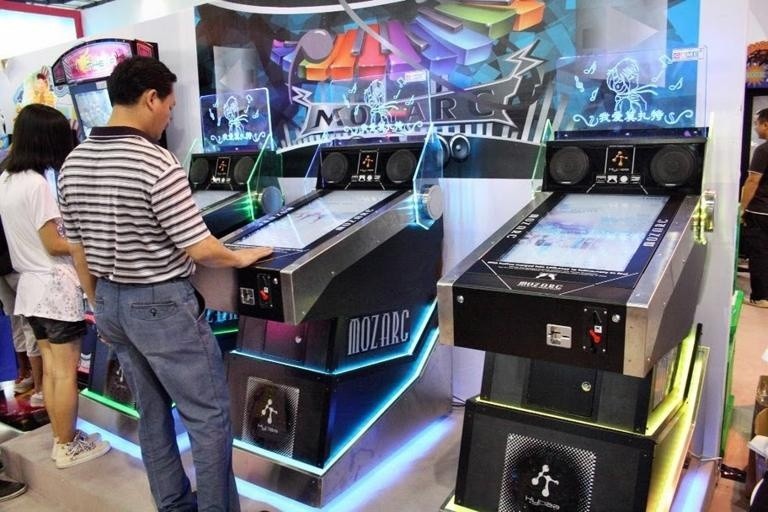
xmin=55 ymin=55 xmax=278 ymax=512
xmin=29 ymin=73 xmax=57 ymax=107
xmin=0 ymin=478 xmax=29 ymax=504
xmin=0 ymin=140 xmax=44 ymax=403
xmin=737 ymin=107 xmax=768 ymax=308
xmin=1 ymin=102 xmax=113 ymax=471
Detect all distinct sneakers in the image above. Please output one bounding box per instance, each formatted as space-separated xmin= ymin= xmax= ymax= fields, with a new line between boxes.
xmin=13 ymin=371 xmax=33 ymax=393
xmin=0 ymin=480 xmax=28 ymax=501
xmin=54 ymin=436 xmax=111 ymax=470
xmin=30 ymin=395 xmax=46 ymax=407
xmin=0 ymin=460 xmax=6 ymax=471
xmin=52 ymin=432 xmax=101 ymax=461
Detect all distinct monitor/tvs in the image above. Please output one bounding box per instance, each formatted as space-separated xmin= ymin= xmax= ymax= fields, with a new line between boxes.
xmin=67 ymin=82 xmax=111 ymax=136
xmin=190 ymin=191 xmax=226 ymax=215
xmin=227 ymin=189 xmax=398 ymax=256
xmin=489 ymin=193 xmax=678 ymax=274
